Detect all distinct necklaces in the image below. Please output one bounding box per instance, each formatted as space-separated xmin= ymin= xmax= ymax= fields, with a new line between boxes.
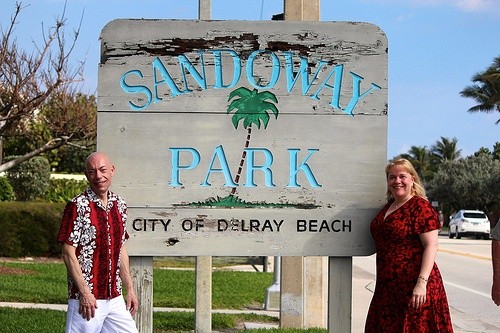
xmin=388 ymin=195 xmax=409 ymax=213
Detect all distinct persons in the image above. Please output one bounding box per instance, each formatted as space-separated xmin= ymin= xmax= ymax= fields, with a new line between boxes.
xmin=57 ymin=152 xmax=138 ymax=333
xmin=364 ymin=158 xmax=454 ymax=333
xmin=439 ymin=211 xmax=443 ymax=228
xmin=489 ymin=218 xmax=500 ymax=307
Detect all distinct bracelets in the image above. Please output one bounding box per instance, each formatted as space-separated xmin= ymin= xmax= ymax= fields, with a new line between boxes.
xmin=419 ymin=276 xmax=428 ymax=282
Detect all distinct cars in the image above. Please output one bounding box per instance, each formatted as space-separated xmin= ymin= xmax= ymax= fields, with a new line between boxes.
xmin=448 ymin=209 xmax=491 ymax=240
